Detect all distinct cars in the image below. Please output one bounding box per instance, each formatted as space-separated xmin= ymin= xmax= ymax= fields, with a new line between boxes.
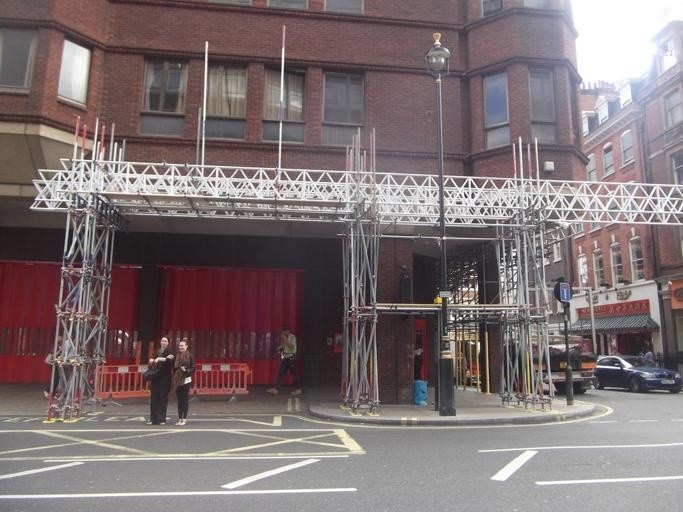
xmin=592 ymin=354 xmax=683 ymax=393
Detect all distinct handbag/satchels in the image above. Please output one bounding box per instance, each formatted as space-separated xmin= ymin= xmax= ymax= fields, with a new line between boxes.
xmin=144 ymin=367 xmax=160 ymax=380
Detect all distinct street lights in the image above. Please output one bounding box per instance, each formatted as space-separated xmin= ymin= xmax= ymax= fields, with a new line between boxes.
xmin=425 ymin=32 xmax=456 ymax=415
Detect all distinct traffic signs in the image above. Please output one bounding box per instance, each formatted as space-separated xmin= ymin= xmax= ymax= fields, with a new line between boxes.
xmin=560 ymin=282 xmax=571 ymax=302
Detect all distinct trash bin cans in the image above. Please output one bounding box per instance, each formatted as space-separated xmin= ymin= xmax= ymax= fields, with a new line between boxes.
xmin=414 ymin=380 xmax=428 ymax=405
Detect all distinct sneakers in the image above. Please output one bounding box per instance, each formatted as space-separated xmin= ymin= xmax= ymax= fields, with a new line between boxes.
xmin=267 ymin=387 xmax=278 ymax=395
xmin=176 ymin=420 xmax=187 ymax=426
xmin=291 ymin=389 xmax=302 ymax=395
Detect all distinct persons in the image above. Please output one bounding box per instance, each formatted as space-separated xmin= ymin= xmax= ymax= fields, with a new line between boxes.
xmin=643 ymin=348 xmax=656 ymax=369
xmin=145 ymin=334 xmax=175 ymax=426
xmin=166 ymin=339 xmax=195 ymax=426
xmin=457 ymin=352 xmax=467 ymax=384
xmin=43 ymin=334 xmax=63 ymax=398
xmin=265 ymin=324 xmax=302 ymax=396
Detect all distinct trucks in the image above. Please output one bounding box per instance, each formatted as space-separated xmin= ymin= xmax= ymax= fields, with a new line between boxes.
xmin=462 ymin=338 xmax=597 ymax=395
